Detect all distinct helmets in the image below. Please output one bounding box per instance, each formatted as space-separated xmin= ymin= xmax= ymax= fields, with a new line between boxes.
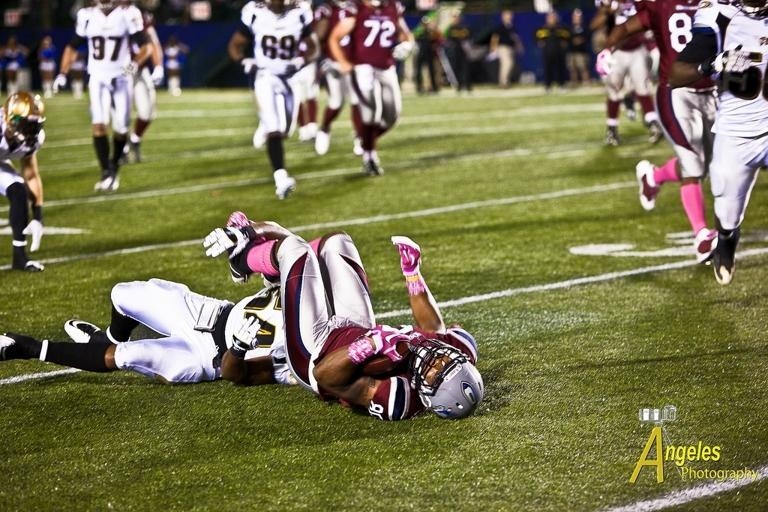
xmin=407 ymin=339 xmax=484 ymax=419
xmin=5 ymin=92 xmax=47 ymax=145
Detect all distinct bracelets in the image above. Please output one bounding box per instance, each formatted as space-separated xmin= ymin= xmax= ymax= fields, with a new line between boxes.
xmin=348 ymin=338 xmax=375 ymax=367
xmin=406 ymin=276 xmax=426 ymax=295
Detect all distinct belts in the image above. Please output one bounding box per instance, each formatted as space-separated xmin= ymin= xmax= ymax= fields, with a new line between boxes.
xmin=216 ymin=305 xmax=235 ymax=354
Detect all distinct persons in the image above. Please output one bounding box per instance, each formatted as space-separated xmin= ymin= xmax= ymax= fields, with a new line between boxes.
xmin=0 ymin=275 xmax=303 ymax=388
xmin=228 ymin=210 xmax=489 ymax=423
xmin=0 ymin=2 xmax=767 ymax=283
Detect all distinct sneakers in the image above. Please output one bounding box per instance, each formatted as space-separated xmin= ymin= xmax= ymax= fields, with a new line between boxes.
xmin=0 ymin=332 xmax=24 ymax=362
xmin=227 ymin=211 xmax=250 ymax=283
xmin=605 ymin=110 xmax=740 ymax=286
xmin=261 ymin=272 xmax=280 ymax=288
xmin=64 ymin=319 xmax=101 ymax=344
xmin=254 ymin=122 xmax=385 ymax=200
xmin=94 ymin=140 xmax=140 ymax=192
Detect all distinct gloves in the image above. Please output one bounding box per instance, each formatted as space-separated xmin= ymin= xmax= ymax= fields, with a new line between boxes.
xmin=713 ymin=49 xmax=751 ymax=72
xmin=392 ymin=42 xmax=413 ymax=59
xmin=364 ymin=325 xmax=411 ymax=362
xmin=54 ymin=74 xmax=68 ymax=94
xmin=281 ymin=57 xmax=304 ymax=75
xmin=319 ymin=58 xmax=340 ymax=77
xmin=243 ymin=58 xmax=263 ymax=73
xmin=202 ymin=226 xmax=256 ymax=259
xmin=391 ymin=236 xmax=421 ymax=276
xmin=115 ymin=66 xmax=137 ymax=79
xmin=230 ymin=316 xmax=262 ymax=358
xmin=22 ymin=219 xmax=44 ymax=253
xmin=151 ymin=68 xmax=163 ymax=85
